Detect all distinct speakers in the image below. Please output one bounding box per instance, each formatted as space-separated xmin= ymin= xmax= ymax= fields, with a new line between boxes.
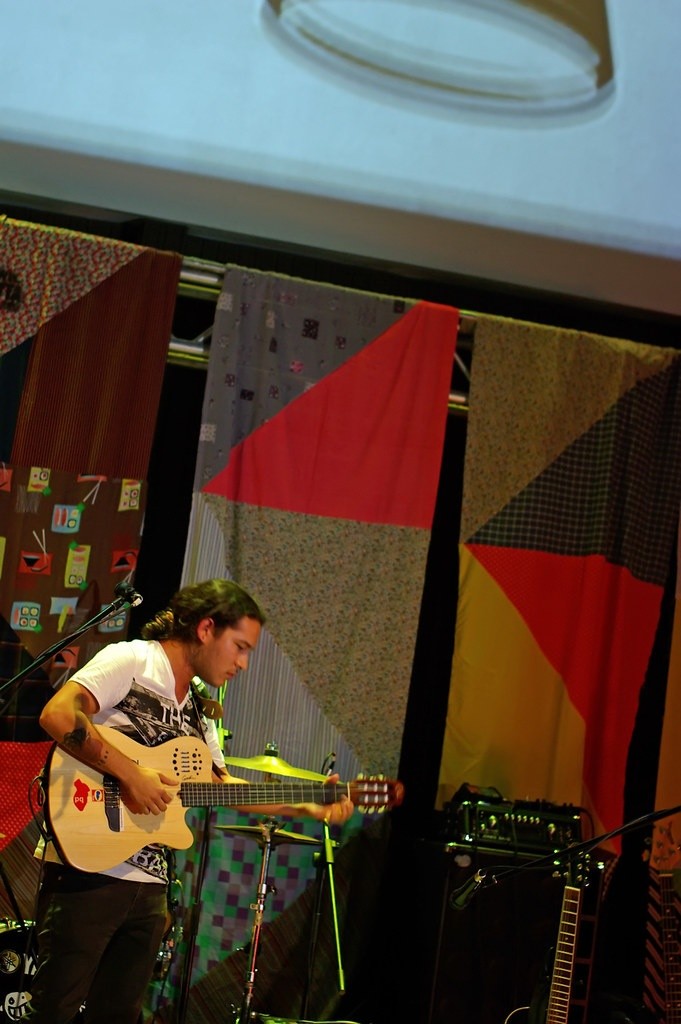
xmin=363 ymin=838 xmax=568 ymax=1024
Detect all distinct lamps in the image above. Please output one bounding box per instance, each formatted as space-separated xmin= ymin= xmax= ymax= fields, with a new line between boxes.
xmin=257 ymin=1 xmax=622 ymax=134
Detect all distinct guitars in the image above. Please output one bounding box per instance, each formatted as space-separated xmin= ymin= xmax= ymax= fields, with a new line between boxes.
xmin=648 ymin=818 xmax=681 ymax=1024
xmin=39 ymin=732 xmax=406 ymax=873
xmin=502 ymin=842 xmax=590 ymax=1024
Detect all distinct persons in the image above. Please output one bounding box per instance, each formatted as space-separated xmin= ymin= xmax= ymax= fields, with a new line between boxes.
xmin=32 ymin=579 xmax=354 ymax=1024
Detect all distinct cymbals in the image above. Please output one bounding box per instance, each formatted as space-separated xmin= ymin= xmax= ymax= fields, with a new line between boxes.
xmin=212 ymin=824 xmax=323 ymax=847
xmin=220 ymin=755 xmax=343 ymax=784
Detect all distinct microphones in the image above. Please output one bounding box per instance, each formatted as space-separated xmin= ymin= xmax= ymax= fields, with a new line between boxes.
xmin=449 ymin=868 xmax=487 ymax=911
xmin=114 ymin=581 xmax=143 ymax=603
